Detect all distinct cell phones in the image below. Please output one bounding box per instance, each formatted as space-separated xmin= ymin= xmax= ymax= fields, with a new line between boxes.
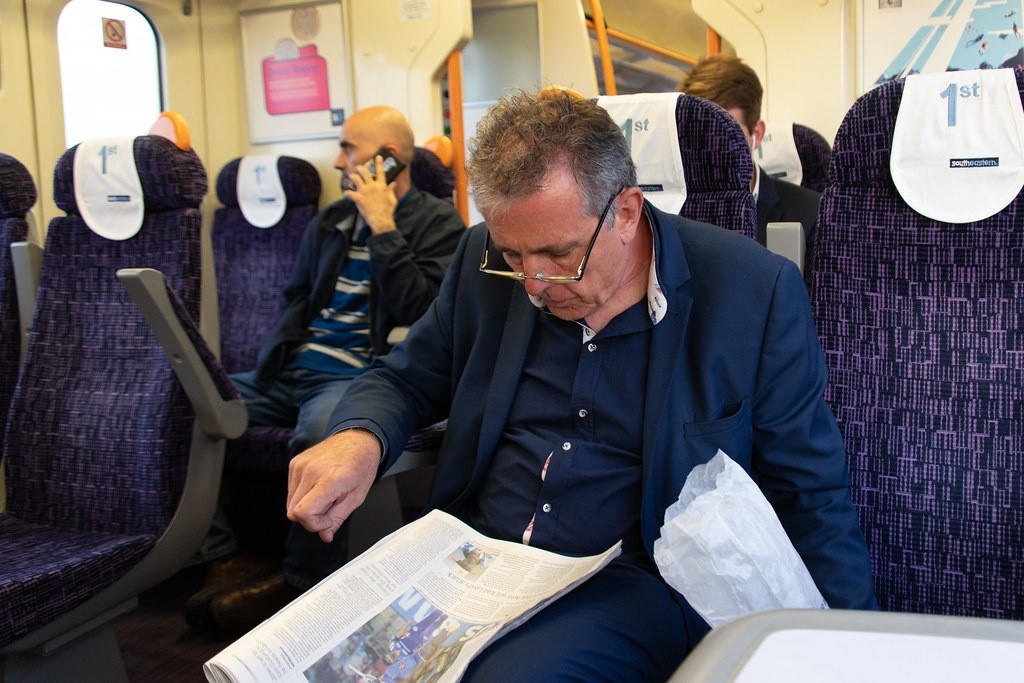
xmin=348 ymin=146 xmax=406 ymax=191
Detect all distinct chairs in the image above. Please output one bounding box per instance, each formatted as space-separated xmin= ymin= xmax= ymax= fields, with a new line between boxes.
xmin=0 ymin=61 xmax=1024 ymax=683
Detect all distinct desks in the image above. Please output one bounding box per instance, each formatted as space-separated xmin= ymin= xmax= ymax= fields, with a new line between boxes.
xmin=664 ymin=609 xmax=1024 ymax=683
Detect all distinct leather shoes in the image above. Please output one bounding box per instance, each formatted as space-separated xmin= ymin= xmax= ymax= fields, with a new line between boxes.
xmin=184 ymin=552 xmax=265 ymax=626
xmin=211 ymin=572 xmax=306 ymax=632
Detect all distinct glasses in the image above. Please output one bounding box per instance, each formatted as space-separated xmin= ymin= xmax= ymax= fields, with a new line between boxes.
xmin=479 ymin=184 xmax=662 ymax=282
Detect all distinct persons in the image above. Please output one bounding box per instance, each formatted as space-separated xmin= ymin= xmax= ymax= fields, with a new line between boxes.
xmin=177 ymin=106 xmax=466 ymax=632
xmin=455 ymin=542 xmax=498 ymax=572
xmin=676 ymin=55 xmax=823 ymax=262
xmin=311 ymin=609 xmax=472 ymax=683
xmin=286 ymin=86 xmax=882 ymax=683
